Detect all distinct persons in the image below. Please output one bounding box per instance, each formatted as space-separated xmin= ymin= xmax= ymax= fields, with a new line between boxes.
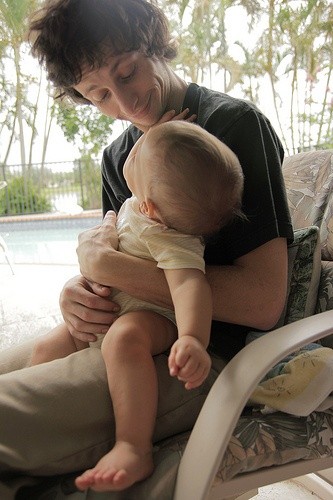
xmin=0 ymin=1 xmax=298 ymax=498
xmin=22 ymin=109 xmax=244 ymax=489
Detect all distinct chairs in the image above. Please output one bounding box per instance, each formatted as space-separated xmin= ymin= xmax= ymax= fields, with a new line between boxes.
xmin=40 ymin=150 xmax=333 ymax=500
